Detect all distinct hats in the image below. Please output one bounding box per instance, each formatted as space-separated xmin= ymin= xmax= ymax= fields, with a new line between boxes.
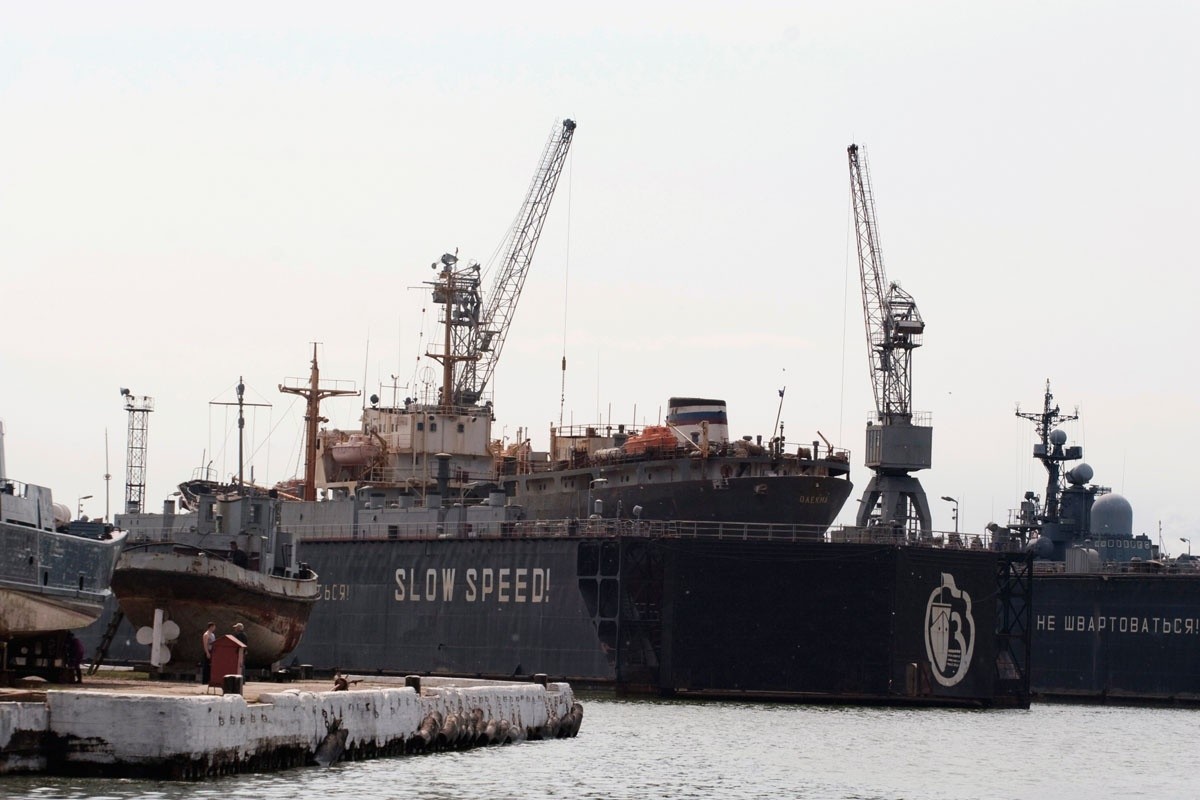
xmin=233 ymin=623 xmax=245 ymax=631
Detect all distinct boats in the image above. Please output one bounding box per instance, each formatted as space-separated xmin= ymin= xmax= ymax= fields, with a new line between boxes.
xmin=0 ymin=581 xmax=101 ymax=681
xmin=111 ymin=473 xmax=322 ymax=667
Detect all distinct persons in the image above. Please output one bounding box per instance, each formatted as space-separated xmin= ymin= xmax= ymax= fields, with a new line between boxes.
xmin=871 ymin=518 xmax=882 ymax=527
xmin=202 ymin=622 xmax=216 ymax=684
xmin=971 ymin=535 xmax=982 ymax=548
xmin=233 ymin=623 xmax=248 ymax=655
xmin=230 ymin=542 xmax=248 ymax=569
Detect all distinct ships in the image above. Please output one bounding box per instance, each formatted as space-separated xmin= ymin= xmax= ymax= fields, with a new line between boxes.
xmin=120 ymin=248 xmax=1200 ymax=710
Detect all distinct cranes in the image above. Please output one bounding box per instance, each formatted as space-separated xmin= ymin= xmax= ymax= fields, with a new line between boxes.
xmin=845 ymin=145 xmax=926 ymax=427
xmin=453 ymin=118 xmax=579 ymax=400
